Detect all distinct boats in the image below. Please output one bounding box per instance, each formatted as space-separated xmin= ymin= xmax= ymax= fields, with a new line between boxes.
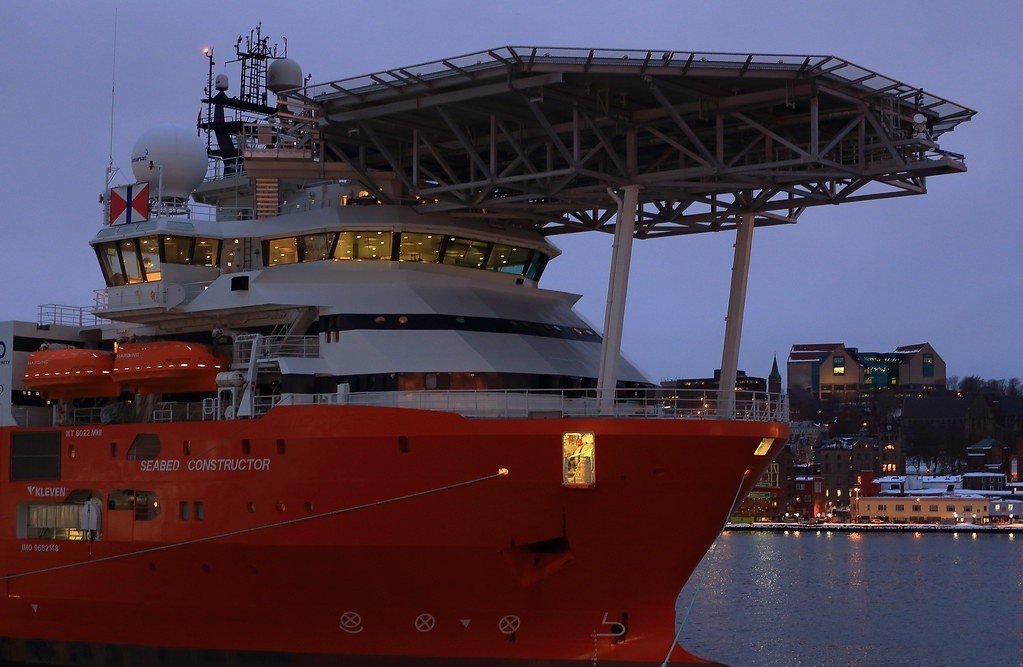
xmin=0 ymin=8 xmax=979 ymax=667
xmin=111 ymin=341 xmax=229 ymax=394
xmin=24 ymin=341 xmax=121 ymax=401
